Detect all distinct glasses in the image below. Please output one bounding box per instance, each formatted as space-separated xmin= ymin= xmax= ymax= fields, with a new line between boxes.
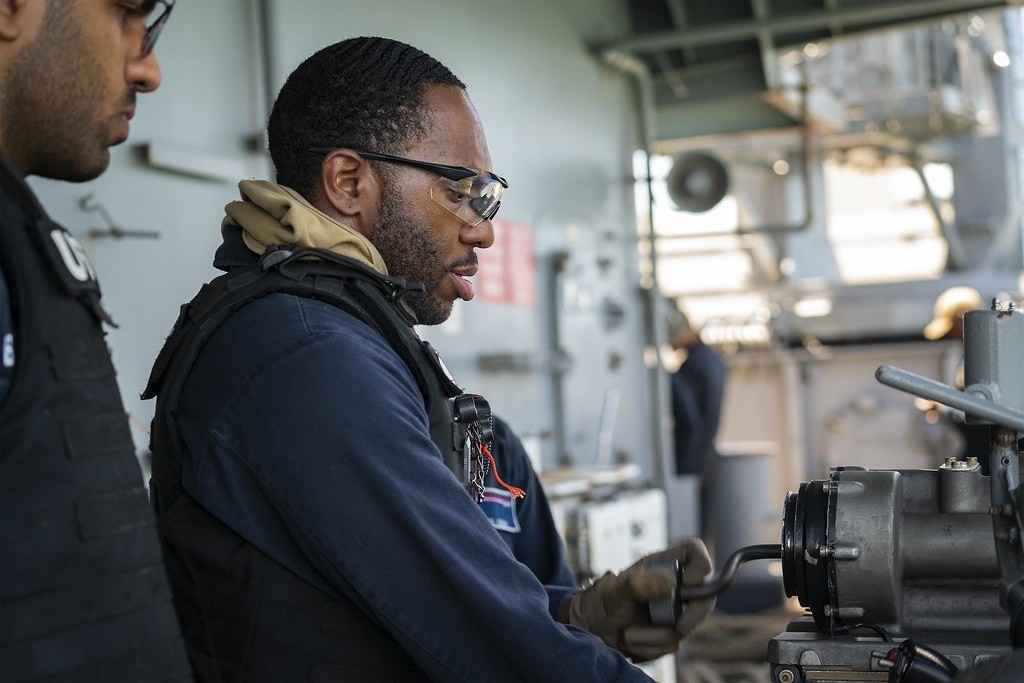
xmin=136 ymin=0 xmax=177 ymax=61
xmin=308 ymin=142 xmax=509 ymax=228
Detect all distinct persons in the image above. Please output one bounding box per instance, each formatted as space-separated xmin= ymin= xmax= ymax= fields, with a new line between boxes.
xmin=0 ymin=0 xmax=193 ymax=683
xmin=140 ymin=35 xmax=718 ymax=683
xmin=669 ymin=308 xmax=728 ymax=539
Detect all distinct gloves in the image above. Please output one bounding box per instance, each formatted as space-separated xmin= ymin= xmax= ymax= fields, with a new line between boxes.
xmin=556 ymin=539 xmax=717 ymax=662
